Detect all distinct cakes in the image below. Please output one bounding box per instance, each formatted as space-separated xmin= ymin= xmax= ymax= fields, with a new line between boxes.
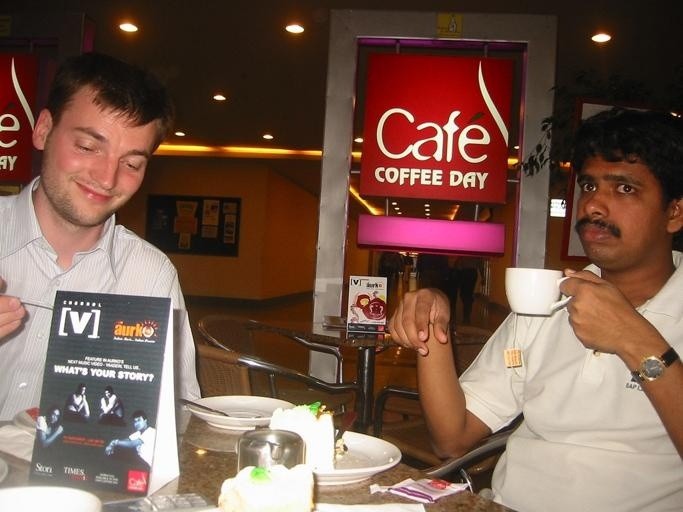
xmin=269 ymin=402 xmax=334 ymax=467
xmin=217 ymin=466 xmax=314 ymax=512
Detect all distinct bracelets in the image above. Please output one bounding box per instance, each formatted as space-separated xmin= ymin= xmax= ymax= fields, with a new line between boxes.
xmin=114 ymin=439 xmax=119 ymax=446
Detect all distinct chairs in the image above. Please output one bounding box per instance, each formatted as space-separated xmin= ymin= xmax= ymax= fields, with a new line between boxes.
xmin=190 ymin=311 xmax=523 ymax=493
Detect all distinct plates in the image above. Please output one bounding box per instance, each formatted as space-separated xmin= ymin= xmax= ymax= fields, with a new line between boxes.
xmin=184 ymin=394 xmax=298 ymax=434
xmin=297 ymin=428 xmax=403 ymax=488
xmin=0 ymin=485 xmax=103 ymax=511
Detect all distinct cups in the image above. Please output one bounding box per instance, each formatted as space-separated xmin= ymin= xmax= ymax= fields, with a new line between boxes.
xmin=503 ymin=265 xmax=575 ymax=318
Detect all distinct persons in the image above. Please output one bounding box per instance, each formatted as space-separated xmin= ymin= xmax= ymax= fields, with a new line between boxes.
xmin=386 ymin=106 xmax=682 ymax=512
xmin=33 ymin=404 xmax=64 ymax=456
xmin=62 ymin=383 xmax=92 ymax=423
xmin=0 ymin=52 xmax=203 ymax=423
xmin=376 ymin=250 xmax=486 ymax=325
xmin=105 ymin=409 xmax=157 ymax=475
xmin=98 ymin=385 xmax=128 ymax=427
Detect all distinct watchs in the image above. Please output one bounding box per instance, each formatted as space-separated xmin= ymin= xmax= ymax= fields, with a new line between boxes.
xmin=631 ymin=346 xmax=680 ymax=388
